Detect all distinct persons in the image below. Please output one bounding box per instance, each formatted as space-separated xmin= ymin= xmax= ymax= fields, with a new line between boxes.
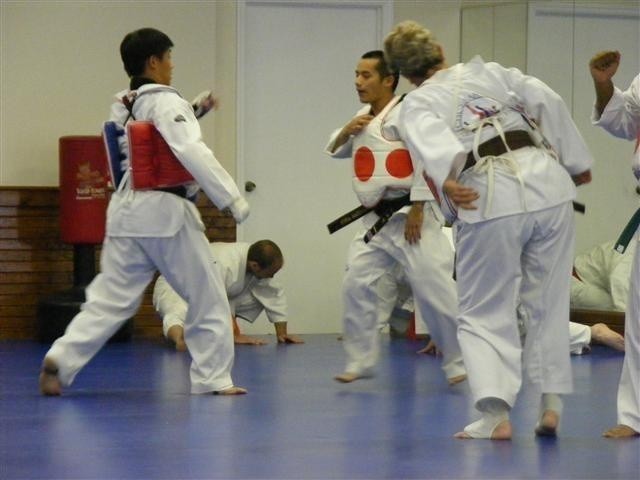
xmin=324 ymin=50 xmax=467 ymax=386
xmin=418 ymin=296 xmax=625 ymax=355
xmin=38 ymin=27 xmax=251 ymax=396
xmin=587 ymin=49 xmax=640 ymax=440
xmin=153 ymin=240 xmax=304 ymax=352
xmin=571 ymin=236 xmax=636 ymax=312
xmin=383 ymin=20 xmax=591 ymax=442
